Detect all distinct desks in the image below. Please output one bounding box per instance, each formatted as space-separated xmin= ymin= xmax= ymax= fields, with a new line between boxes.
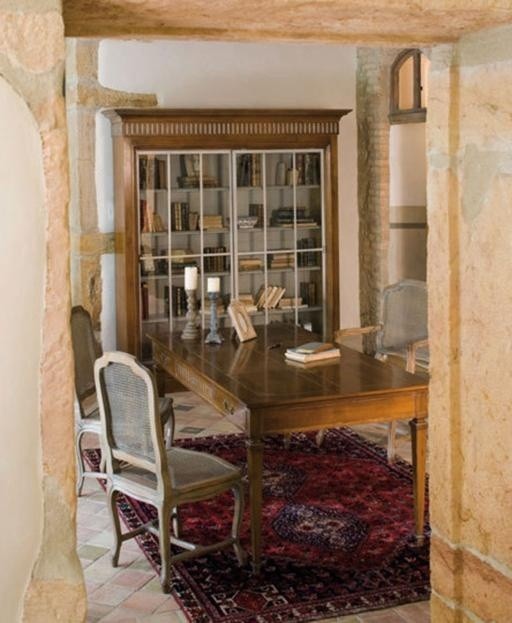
xmin=136 ymin=325 xmax=441 ymax=577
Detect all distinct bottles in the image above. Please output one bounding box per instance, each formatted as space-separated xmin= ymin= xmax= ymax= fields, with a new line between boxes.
xmin=277 ymin=163 xmax=299 ymax=186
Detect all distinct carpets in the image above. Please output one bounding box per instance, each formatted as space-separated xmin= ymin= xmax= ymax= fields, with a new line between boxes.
xmin=80 ymin=428 xmax=436 ymax=621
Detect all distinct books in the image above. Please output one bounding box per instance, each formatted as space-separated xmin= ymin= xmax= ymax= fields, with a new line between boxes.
xmin=140 ymin=153 xmax=320 ymax=319
xmin=284 ymin=342 xmax=341 ymax=364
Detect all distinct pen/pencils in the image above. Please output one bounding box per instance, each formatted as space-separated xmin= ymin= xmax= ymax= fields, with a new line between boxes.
xmin=269 ymin=343 xmax=281 ymax=350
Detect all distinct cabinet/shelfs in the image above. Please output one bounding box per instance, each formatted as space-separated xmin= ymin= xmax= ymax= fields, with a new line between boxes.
xmin=103 ymin=102 xmax=355 ymax=401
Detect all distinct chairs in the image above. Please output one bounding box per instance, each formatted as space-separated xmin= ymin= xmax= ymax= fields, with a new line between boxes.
xmin=71 ymin=305 xmax=177 ymax=498
xmin=312 ymin=276 xmax=428 ymax=466
xmin=93 ymin=350 xmax=244 ymax=597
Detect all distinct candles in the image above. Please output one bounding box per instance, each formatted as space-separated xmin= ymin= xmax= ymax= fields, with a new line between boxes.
xmin=182 ymin=267 xmax=198 ymax=291
xmin=207 ymin=277 xmax=220 ymax=294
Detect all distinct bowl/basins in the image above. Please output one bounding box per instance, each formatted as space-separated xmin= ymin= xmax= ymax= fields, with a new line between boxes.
xmin=237 ymin=215 xmax=259 ymax=229
